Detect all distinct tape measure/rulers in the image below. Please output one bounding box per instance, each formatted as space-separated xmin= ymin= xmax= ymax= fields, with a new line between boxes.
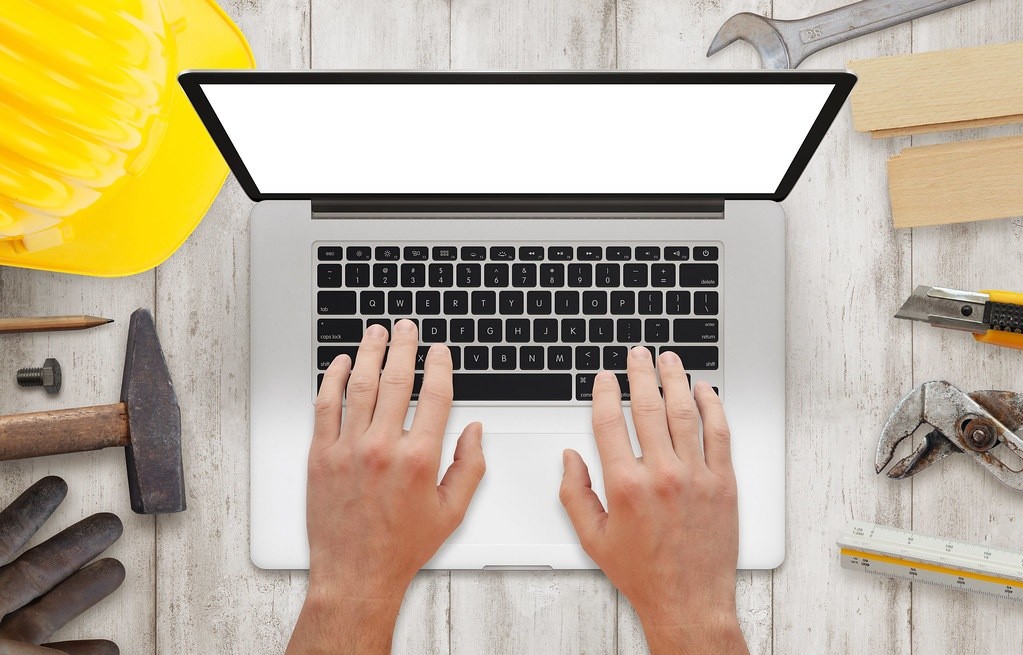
xmin=836 ymin=518 xmax=1023 ymax=602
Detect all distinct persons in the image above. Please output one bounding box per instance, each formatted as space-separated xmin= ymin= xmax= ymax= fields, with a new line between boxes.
xmin=285 ymin=318 xmax=751 ymax=655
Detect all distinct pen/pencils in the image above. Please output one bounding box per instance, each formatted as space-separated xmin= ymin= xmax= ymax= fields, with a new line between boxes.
xmin=0 ymin=314 xmax=114 ymax=334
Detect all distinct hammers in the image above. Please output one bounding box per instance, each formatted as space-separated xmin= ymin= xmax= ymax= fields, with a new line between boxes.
xmin=0 ymin=305 xmax=188 ymax=514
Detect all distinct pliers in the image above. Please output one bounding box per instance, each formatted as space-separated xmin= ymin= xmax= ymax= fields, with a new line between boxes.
xmin=873 ymin=376 xmax=1023 ymax=491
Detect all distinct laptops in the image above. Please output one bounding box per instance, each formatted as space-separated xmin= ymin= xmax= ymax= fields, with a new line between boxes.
xmin=178 ymin=69 xmax=857 ymax=571
